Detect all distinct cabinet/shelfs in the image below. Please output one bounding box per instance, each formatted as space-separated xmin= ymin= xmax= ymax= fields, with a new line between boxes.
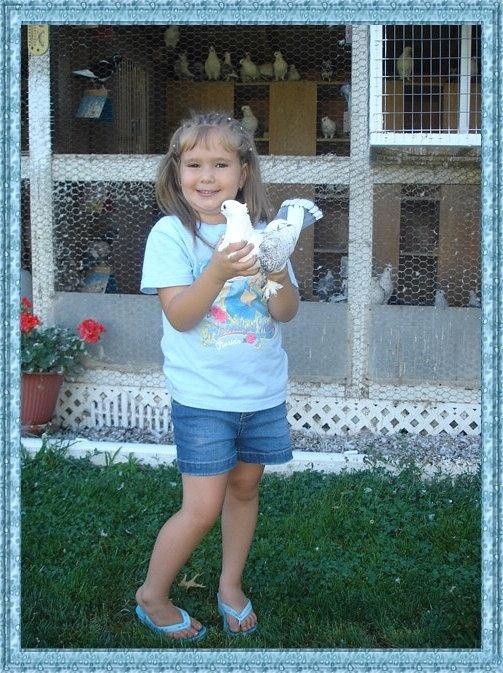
xmin=158 ymin=73 xmax=481 ymax=308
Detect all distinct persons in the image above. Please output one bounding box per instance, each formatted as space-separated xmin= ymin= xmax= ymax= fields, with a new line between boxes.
xmin=135 ymin=110 xmax=300 ymax=644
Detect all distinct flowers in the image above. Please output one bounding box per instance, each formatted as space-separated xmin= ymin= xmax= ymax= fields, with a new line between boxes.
xmin=19 ymin=295 xmax=105 ymax=382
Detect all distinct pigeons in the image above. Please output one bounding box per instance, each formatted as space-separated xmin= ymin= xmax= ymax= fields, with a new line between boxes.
xmin=219 ymin=199 xmax=324 ymax=303
xmin=241 ymin=106 xmax=259 ymax=136
xmin=88 ymin=240 xmax=112 ymax=263
xmin=321 ymin=116 xmax=336 ymax=141
xmin=163 ymin=26 xmax=351 ymax=102
xmin=396 ymin=47 xmax=478 ymax=81
xmin=316 ymin=256 xmax=481 ymax=307
xmin=74 ymin=53 xmax=126 ymax=84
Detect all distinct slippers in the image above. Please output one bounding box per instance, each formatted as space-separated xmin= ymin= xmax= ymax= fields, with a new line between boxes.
xmin=135 ymin=604 xmax=206 ymax=642
xmin=216 ymin=589 xmax=257 ymax=636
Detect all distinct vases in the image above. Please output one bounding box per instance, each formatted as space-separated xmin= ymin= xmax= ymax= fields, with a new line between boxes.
xmin=21 ymin=369 xmax=66 ymax=436
